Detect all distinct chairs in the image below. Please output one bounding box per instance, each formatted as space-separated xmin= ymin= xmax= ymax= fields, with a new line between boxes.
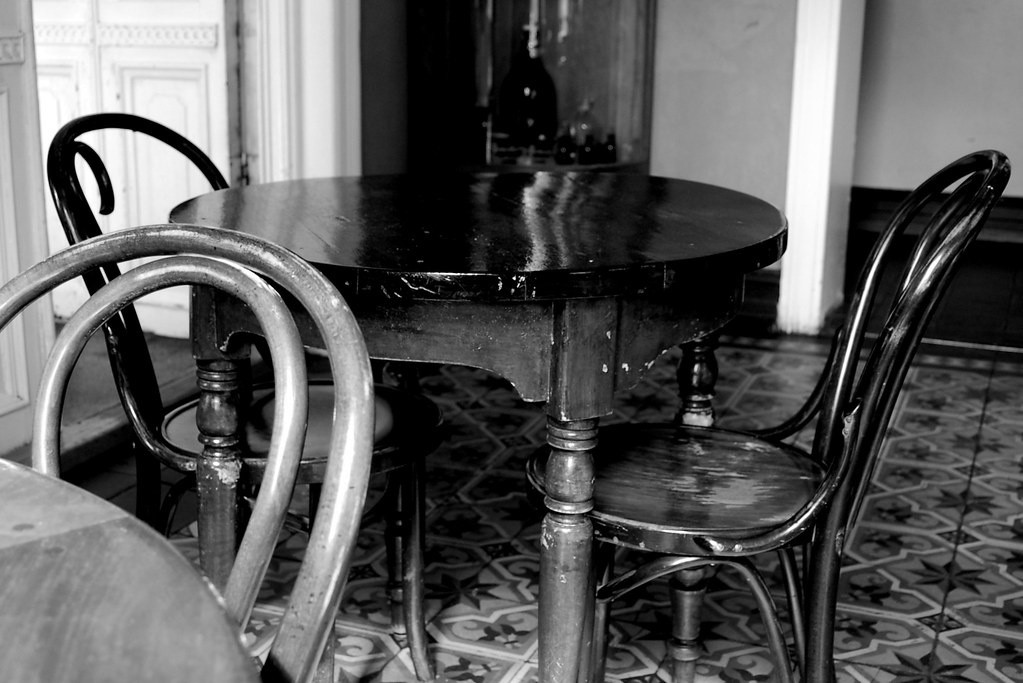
xmin=47 ymin=110 xmax=437 ymax=682
xmin=525 ymin=149 xmax=1012 ymax=683
xmin=0 ymin=225 xmax=375 ymax=683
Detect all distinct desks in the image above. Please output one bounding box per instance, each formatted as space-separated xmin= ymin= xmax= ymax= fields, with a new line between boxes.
xmin=165 ymin=171 xmax=788 ymax=683
xmin=0 ymin=457 xmax=258 ymax=683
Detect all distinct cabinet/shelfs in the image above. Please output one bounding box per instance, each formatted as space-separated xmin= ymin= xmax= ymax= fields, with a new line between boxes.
xmin=405 ymin=0 xmax=661 ymax=170
